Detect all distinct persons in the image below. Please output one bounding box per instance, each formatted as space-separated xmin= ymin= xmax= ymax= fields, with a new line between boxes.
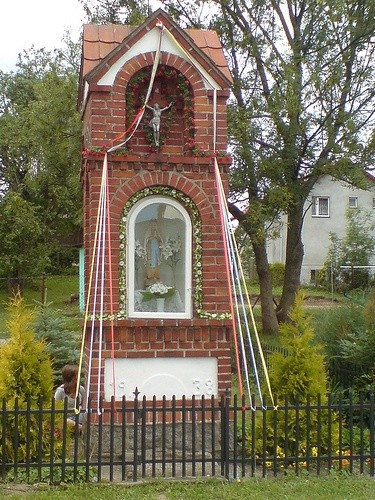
xmin=143 ymin=102 xmax=171 ymax=148
xmin=53 ymin=366 xmax=85 ymax=434
xmin=143 ymin=219 xmax=162 ymax=286
